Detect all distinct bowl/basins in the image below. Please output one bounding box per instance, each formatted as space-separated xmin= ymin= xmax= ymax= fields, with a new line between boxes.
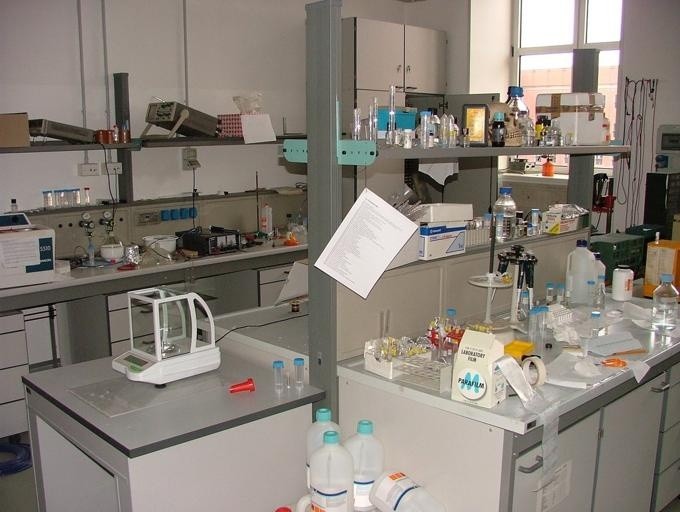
xmin=99 ymin=243 xmax=125 ymax=262
xmin=142 ymin=234 xmax=180 ymax=252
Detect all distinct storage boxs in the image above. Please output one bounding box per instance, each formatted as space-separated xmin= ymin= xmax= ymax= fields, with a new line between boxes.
xmin=0 ymin=111 xmax=30 ymax=148
xmin=537 ymin=91 xmax=605 ymax=144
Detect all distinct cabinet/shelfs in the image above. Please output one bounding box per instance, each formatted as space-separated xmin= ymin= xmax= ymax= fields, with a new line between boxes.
xmin=341 ymin=16 xmax=498 ymax=229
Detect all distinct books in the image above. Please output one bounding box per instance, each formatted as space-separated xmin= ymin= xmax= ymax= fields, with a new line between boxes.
xmin=312 ymin=183 xmax=418 ymax=302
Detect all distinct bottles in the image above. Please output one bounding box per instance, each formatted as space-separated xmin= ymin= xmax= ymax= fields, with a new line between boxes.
xmin=518 ymin=274 xmax=608 ymax=353
xmin=593 ymin=251 xmax=606 ymax=275
xmin=650 ymin=273 xmax=679 ymax=330
xmin=271 ymin=361 xmax=286 ymax=387
xmin=350 ymin=83 xmax=575 ymax=151
xmin=292 ymin=223 xmax=301 ymax=241
xmin=260 ymin=203 xmax=273 ymax=232
xmin=603 ymin=113 xmax=611 ymax=145
xmin=120 ymin=125 xmax=129 ymax=144
xmin=446 ymin=308 xmax=459 ymax=327
xmin=95 ymin=124 xmax=120 ymax=145
xmin=294 ymin=407 xmax=446 ymax=512
xmin=612 ymin=265 xmax=633 ymax=303
xmin=293 ymin=358 xmax=306 ymax=383
xmin=432 ymin=328 xmax=464 ymax=365
xmin=43 ymin=187 xmax=91 ymax=210
xmin=285 ymin=213 xmax=293 ymax=232
xmin=542 ymin=158 xmax=554 ymax=176
xmin=483 ymin=186 xmax=540 ymax=243
xmin=11 ymin=199 xmax=16 ymax=213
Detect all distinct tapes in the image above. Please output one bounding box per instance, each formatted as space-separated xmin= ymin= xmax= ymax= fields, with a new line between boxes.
xmin=521 ymin=357 xmax=546 ymax=388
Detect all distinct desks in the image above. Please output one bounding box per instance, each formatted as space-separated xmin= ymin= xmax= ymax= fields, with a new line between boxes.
xmin=337 ymin=291 xmax=680 ymax=511
xmin=0 ymin=229 xmax=309 ymax=441
xmin=21 ymin=341 xmax=326 ymax=511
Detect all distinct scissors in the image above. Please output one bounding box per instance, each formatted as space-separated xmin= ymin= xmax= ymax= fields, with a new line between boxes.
xmin=595 ymin=358 xmax=626 ymax=367
